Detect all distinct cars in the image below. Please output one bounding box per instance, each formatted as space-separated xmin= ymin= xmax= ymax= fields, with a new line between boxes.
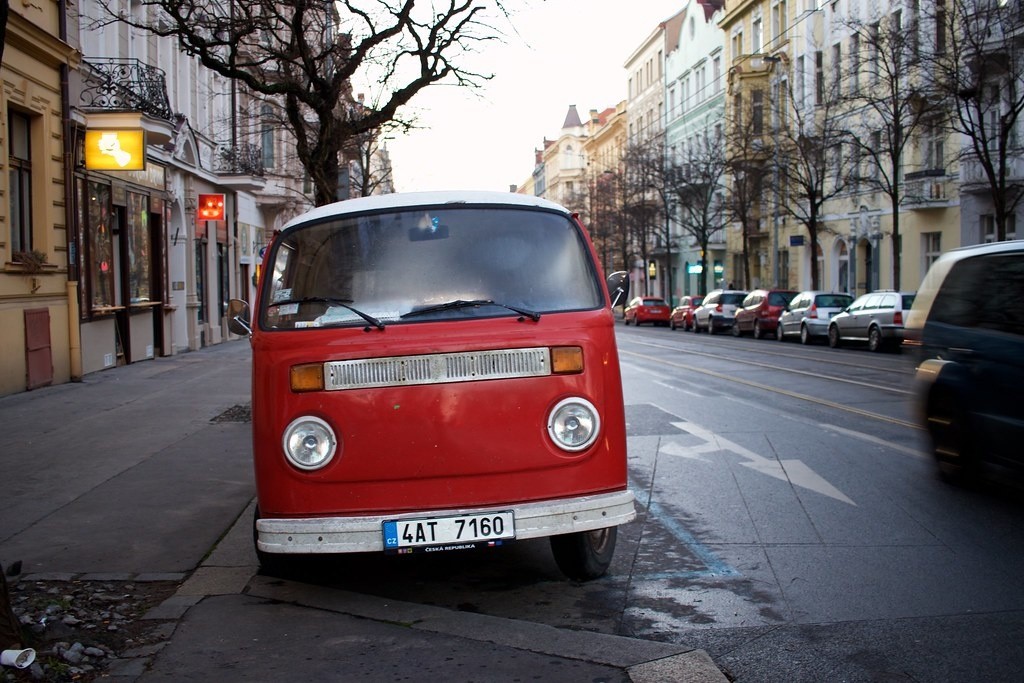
xmin=624 ymin=296 xmax=671 ymax=327
xmin=828 ymin=287 xmax=917 ymax=352
xmin=777 ymin=291 xmax=855 ymax=346
xmin=733 ymin=288 xmax=800 ymax=339
xmin=670 ymin=295 xmax=706 ymax=333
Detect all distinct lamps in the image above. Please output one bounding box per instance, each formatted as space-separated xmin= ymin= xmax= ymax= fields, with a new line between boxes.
xmin=762 ymin=57 xmax=778 ymax=62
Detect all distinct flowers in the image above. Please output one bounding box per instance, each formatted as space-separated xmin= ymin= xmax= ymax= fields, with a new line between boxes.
xmin=15 ymin=247 xmax=47 ymax=273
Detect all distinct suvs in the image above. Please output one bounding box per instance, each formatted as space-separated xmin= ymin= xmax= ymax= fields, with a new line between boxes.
xmin=691 ymin=288 xmax=750 ymax=335
xmin=908 ymin=238 xmax=1023 ymax=499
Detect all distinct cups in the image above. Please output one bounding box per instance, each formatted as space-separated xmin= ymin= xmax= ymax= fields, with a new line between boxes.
xmin=0 ymin=648 xmax=36 ymax=669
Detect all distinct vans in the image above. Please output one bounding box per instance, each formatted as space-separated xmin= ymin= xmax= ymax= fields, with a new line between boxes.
xmin=226 ymin=189 xmax=638 ymax=583
